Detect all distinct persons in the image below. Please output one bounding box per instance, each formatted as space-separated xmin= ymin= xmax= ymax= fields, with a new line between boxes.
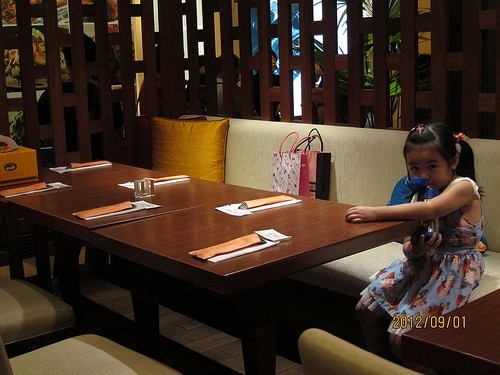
xmin=138 ymin=40 xmax=244 ymax=126
xmin=251 ymin=47 xmax=291 ymax=121
xmin=32 ymin=34 xmax=132 ymax=280
xmin=343 ymin=119 xmax=487 ymax=361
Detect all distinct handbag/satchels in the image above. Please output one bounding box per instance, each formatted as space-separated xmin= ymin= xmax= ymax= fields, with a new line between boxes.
xmin=271 ymin=132 xmax=311 ymax=197
xmin=294 ymin=128 xmax=331 ymax=201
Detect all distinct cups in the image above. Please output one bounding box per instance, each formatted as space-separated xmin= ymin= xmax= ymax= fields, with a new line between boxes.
xmin=134 ymin=178 xmax=155 ymax=199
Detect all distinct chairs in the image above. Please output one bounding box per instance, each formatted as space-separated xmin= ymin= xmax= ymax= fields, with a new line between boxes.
xmin=0 ymin=278 xmax=75 ymax=346
xmin=0 ymin=332 xmax=185 ymax=375
xmin=299 ymin=327 xmax=425 ymax=375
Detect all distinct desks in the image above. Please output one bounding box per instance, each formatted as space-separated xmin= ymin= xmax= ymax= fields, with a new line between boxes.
xmin=0 ymin=160 xmax=500 ymax=375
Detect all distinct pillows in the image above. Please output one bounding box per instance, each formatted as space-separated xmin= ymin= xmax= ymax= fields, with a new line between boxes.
xmin=134 ymin=116 xmax=230 ymax=184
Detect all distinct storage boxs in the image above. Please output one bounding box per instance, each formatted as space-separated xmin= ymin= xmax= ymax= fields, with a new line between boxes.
xmin=0 ymin=145 xmax=39 ymax=186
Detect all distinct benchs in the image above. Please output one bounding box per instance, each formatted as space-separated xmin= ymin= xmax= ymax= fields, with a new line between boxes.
xmin=130 ymin=113 xmax=500 ymax=301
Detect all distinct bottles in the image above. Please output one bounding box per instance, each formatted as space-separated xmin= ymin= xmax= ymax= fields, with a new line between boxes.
xmin=407 ymin=176 xmax=440 ymax=238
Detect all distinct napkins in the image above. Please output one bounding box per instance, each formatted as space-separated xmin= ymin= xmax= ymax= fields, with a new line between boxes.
xmin=214 ymin=195 xmax=302 ymax=217
xmin=84 ymin=200 xmax=160 ymax=220
xmin=5 ymin=182 xmax=71 ymax=198
xmin=117 ymin=177 xmax=191 ymax=191
xmin=49 ymin=161 xmax=112 ymax=174
xmin=189 ymin=227 xmax=291 ymax=264
